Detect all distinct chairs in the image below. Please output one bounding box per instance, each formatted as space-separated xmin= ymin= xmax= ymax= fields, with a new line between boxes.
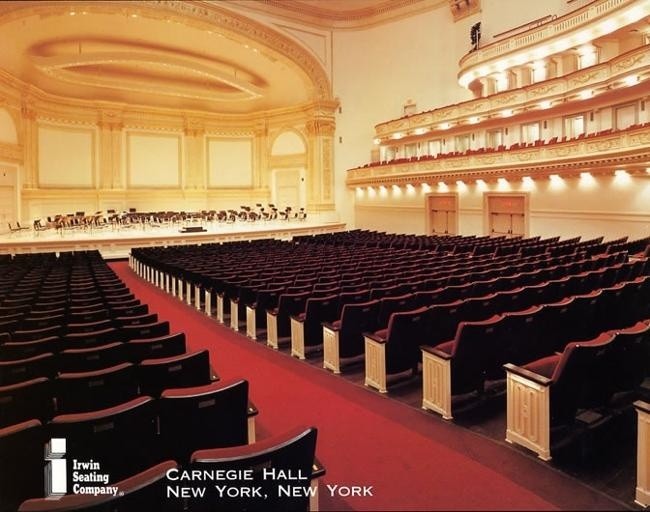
xmin=0 ymin=227 xmax=648 ymax=512
xmin=8 ymin=203 xmax=309 ymax=237
